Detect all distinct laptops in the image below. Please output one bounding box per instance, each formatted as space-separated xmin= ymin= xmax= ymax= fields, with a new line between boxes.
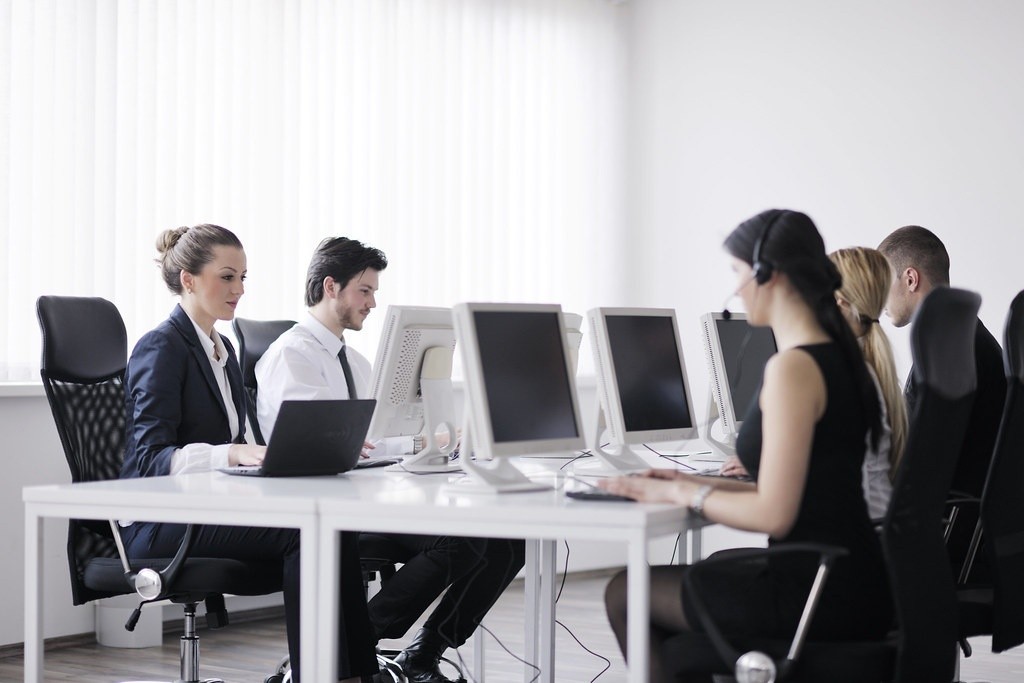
xmin=217 ymin=399 xmax=377 ymax=478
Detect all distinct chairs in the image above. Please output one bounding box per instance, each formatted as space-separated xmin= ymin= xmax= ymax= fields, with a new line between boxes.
xmin=232 ymin=316 xmax=468 ymax=683
xmin=659 ymin=286 xmax=979 ymax=683
xmin=35 ymin=294 xmax=282 ymax=683
xmin=870 ymin=291 xmax=1024 ymax=657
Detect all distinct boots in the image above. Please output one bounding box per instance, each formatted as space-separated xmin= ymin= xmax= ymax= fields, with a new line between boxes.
xmin=393 ymin=626 xmax=464 ymax=683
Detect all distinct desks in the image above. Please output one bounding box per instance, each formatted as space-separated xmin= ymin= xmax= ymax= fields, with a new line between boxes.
xmin=315 ymin=456 xmax=723 ymax=683
xmin=22 ymin=446 xmax=659 ymax=683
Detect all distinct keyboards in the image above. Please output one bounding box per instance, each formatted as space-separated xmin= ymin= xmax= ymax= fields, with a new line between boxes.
xmin=697 ymin=467 xmax=755 ymax=482
xmin=566 ymin=487 xmax=635 ymax=502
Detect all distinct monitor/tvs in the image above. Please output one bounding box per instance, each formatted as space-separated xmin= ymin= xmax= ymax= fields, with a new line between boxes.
xmin=592 ymin=307 xmax=699 ymax=475
xmin=697 ymin=311 xmax=777 ymax=462
xmin=365 ymin=304 xmax=462 ymax=474
xmin=457 ymin=301 xmax=587 ymax=494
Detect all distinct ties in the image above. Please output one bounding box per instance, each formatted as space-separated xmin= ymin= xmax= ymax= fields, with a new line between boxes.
xmin=338 ymin=346 xmax=358 ymax=401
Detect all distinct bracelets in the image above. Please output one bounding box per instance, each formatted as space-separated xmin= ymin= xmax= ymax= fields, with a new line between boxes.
xmin=693 ymin=484 xmax=717 ymax=519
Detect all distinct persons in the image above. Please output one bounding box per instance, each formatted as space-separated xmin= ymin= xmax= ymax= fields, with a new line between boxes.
xmin=115 ymin=222 xmax=379 ymax=683
xmin=826 ymin=225 xmax=1007 ymax=613
xmin=253 ymin=234 xmax=525 ymax=683
xmin=586 ymin=209 xmax=898 ymax=683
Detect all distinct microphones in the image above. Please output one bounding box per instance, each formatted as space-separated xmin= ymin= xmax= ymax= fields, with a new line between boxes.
xmin=722 ymin=272 xmax=756 ymax=320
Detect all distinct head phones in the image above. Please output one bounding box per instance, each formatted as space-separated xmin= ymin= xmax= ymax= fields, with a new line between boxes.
xmin=753 ymin=209 xmax=791 ymax=283
